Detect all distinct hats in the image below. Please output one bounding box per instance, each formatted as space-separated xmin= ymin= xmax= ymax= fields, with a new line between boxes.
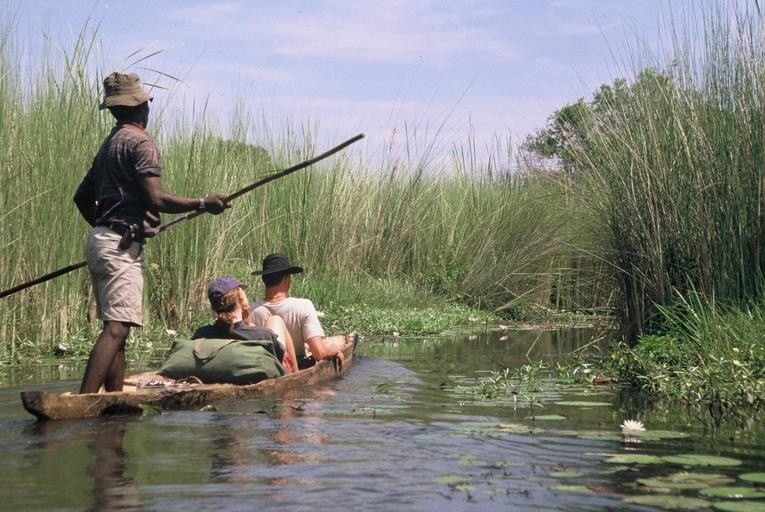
xmin=250 ymin=254 xmax=303 ymax=275
xmin=208 ymin=276 xmax=248 ymax=296
xmin=99 ymin=73 xmax=153 ymax=110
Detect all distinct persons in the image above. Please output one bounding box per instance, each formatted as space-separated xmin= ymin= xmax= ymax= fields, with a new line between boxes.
xmin=189 ymin=275 xmax=300 ymax=374
xmin=70 ymin=71 xmax=236 ymax=397
xmin=244 ymin=252 xmax=344 ymax=373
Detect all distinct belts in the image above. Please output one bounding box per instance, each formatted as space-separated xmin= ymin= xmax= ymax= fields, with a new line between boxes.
xmin=96 ymin=217 xmax=144 ymax=246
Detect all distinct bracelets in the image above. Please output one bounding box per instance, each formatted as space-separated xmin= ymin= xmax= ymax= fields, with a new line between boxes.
xmin=197 ymin=197 xmax=207 ymax=214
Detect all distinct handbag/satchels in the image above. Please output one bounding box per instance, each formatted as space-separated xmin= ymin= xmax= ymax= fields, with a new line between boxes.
xmin=156 ymin=338 xmax=287 ymax=385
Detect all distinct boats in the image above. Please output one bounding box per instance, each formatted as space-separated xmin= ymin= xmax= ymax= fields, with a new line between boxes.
xmin=20 ymin=331 xmax=359 ymax=420
